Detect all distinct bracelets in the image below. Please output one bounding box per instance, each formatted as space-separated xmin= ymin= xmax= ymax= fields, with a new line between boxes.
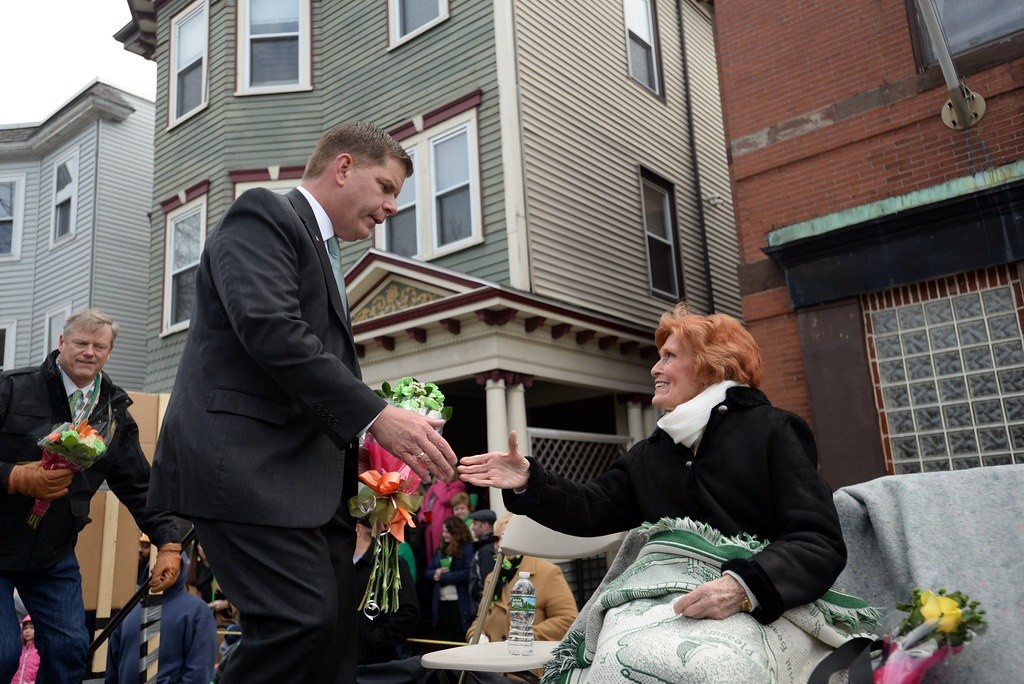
xmin=737 ymin=580 xmax=752 ymax=612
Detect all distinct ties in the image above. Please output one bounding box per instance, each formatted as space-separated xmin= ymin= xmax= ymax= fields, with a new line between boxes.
xmin=328 ymin=236 xmax=347 ymax=321
xmin=69 ymin=389 xmax=83 ymax=418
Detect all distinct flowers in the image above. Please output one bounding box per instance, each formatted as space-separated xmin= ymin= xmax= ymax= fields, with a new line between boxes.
xmin=873 ymin=582 xmax=986 ymax=684
xmin=348 ymin=376 xmax=447 ymax=611
xmin=28 ymin=415 xmax=108 ymax=528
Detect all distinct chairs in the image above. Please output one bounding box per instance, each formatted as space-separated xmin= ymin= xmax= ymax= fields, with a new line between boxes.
xmin=420 ymin=514 xmax=629 ymax=684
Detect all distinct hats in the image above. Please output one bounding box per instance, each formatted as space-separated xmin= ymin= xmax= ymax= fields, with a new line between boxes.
xmin=468 ymin=509 xmax=496 ymax=524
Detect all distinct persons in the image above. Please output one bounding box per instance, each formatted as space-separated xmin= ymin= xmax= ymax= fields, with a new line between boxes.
xmin=146 ymin=123 xmax=458 ymax=684
xmin=352 ymin=459 xmax=578 ymax=684
xmin=104 ymin=533 xmax=242 ymax=684
xmin=457 ymin=304 xmax=847 ymax=684
xmin=0 ymin=308 xmax=181 ymax=684
xmin=11 ymin=615 xmax=40 ymax=684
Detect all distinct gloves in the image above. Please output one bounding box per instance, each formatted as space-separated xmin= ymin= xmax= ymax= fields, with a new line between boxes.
xmin=469 ymin=633 xmax=489 ymax=645
xmin=150 ymin=543 xmax=182 ymax=592
xmin=8 ymin=460 xmax=73 ymax=497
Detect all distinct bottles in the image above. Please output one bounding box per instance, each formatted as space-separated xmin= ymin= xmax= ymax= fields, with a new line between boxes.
xmin=508 ymin=572 xmax=536 ymax=655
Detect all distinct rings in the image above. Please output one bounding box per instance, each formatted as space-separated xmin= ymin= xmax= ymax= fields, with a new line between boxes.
xmin=416 ymin=451 xmax=425 ymax=460
